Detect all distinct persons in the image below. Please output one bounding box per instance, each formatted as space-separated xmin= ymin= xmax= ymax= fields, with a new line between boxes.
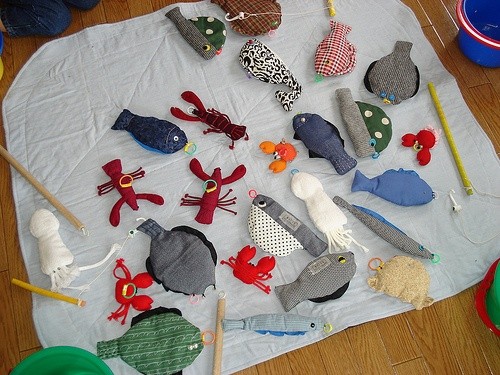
xmin=0 ymin=0 xmax=100 ymax=37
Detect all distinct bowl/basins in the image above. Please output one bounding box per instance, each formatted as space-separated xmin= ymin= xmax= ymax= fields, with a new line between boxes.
xmin=9 ymin=345 xmax=114 ymax=375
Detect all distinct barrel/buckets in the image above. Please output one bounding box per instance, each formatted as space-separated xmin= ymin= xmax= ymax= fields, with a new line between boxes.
xmin=454 ymin=0 xmax=500 ymax=68
